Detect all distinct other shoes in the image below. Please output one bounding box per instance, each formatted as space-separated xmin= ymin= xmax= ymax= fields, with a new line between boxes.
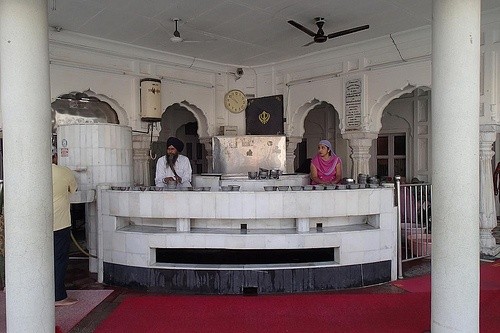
xmin=54 ymin=296 xmax=80 ymax=306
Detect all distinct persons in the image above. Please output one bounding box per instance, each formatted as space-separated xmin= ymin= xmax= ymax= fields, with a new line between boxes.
xmin=309 ymin=140 xmax=342 ymax=185
xmin=154 ymin=137 xmax=193 ymax=187
xmin=52 ymin=145 xmax=79 ymax=306
xmin=493 ymin=162 xmax=500 ymax=203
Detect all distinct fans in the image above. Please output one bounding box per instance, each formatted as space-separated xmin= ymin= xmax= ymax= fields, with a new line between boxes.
xmin=287 ymin=17 xmax=370 ymax=48
xmin=155 ymin=22 xmax=217 ymax=51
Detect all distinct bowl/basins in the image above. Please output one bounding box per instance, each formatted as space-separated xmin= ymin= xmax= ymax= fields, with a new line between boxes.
xmin=408 ymin=234 xmax=432 ymax=259
xmin=401 ymin=223 xmax=426 ymax=238
xmin=110 ymin=179 xmax=211 ymax=192
xmin=247 ymin=167 xmax=395 ymax=191
xmin=221 ymin=183 xmax=240 ymax=192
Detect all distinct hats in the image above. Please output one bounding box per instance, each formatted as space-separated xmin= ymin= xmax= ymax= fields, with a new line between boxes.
xmin=166 ymin=137 xmax=183 ymax=151
xmin=318 ymin=140 xmax=332 ymax=147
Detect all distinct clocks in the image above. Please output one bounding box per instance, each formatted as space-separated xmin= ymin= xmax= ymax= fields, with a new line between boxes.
xmin=224 ymin=89 xmax=247 ymax=114
